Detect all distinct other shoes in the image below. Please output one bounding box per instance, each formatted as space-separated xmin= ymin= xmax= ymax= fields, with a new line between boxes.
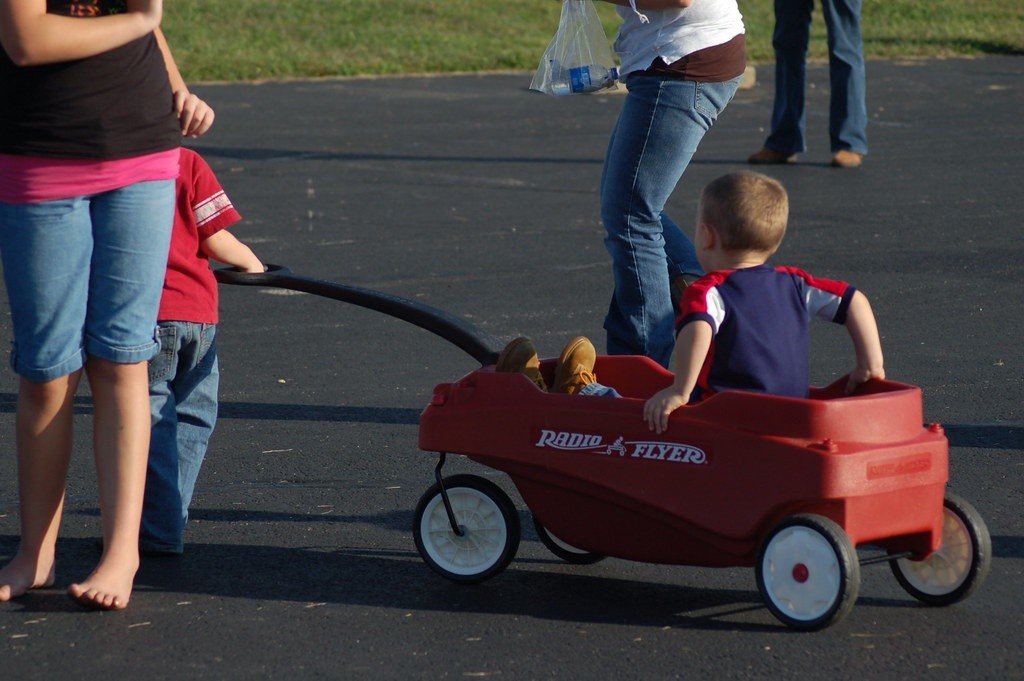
xmin=748 ymin=146 xmax=797 ymax=163
xmin=832 ymin=150 xmax=862 ymax=167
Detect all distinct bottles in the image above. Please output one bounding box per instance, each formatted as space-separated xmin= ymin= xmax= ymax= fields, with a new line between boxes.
xmin=551 ymin=64 xmax=618 ymax=95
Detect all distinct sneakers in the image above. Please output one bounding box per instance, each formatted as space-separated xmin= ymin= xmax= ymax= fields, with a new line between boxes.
xmin=553 ymin=335 xmax=597 ymax=394
xmin=497 ymin=336 xmax=549 ymax=393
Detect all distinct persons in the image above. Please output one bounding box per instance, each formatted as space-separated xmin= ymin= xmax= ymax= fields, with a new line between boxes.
xmin=496 ymin=169 xmax=886 ymax=435
xmin=749 ymin=0 xmax=869 ymax=165
xmin=592 ymin=0 xmax=745 ymax=366
xmin=138 ymin=148 xmax=264 ymax=555
xmin=0 ymin=0 xmax=217 ymax=609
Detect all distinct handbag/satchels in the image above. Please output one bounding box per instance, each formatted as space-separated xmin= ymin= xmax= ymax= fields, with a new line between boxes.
xmin=528 ymin=0 xmax=621 ymax=96
